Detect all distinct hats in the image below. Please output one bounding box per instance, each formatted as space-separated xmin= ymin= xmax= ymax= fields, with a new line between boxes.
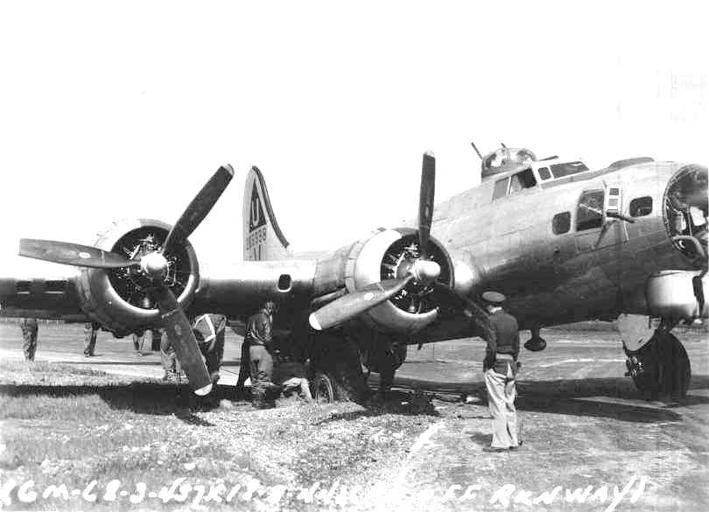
xmin=482 ymin=292 xmax=505 ymax=306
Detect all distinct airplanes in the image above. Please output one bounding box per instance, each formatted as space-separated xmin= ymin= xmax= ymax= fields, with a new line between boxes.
xmin=0 ymin=140 xmax=708 ymax=427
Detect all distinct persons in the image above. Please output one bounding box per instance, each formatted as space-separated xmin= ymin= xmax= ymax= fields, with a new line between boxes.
xmin=244 ymin=300 xmax=280 ymax=409
xmin=83 ymin=323 xmax=97 ymax=357
xmin=160 ymin=329 xmax=220 ymax=384
xmin=20 ymin=316 xmax=38 ymax=362
xmin=482 ymin=292 xmax=520 ymax=453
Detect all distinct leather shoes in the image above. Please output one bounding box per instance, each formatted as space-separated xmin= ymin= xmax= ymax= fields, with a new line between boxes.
xmin=483 ymin=445 xmax=518 ymax=453
